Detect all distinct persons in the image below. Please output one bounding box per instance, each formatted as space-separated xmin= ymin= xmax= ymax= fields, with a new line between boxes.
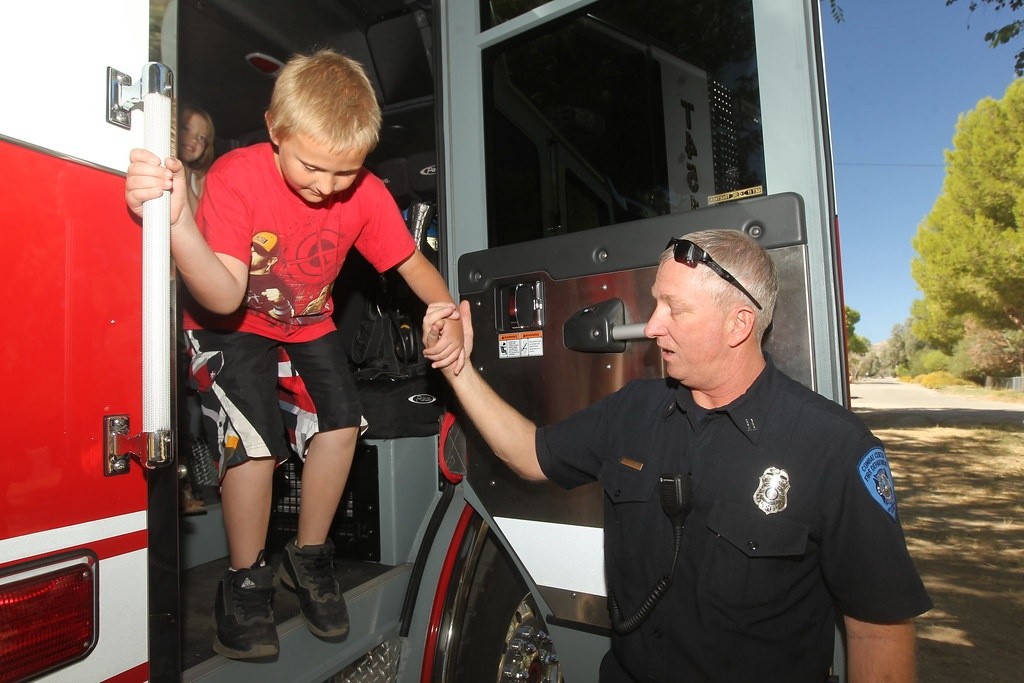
xmin=174 ymin=100 xmax=222 ymax=518
xmin=420 ymin=226 xmax=933 ymax=683
xmin=126 ymin=48 xmax=466 ymax=660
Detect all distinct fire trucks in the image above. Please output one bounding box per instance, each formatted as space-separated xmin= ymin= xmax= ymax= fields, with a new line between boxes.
xmin=1 ymin=0 xmax=853 ymax=682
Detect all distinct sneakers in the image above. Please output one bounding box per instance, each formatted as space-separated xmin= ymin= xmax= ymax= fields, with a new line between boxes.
xmin=211 ymin=537 xmax=349 ymax=660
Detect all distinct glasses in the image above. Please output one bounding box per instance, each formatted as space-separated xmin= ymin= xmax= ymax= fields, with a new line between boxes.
xmin=665 ymin=236 xmax=763 ymax=311
